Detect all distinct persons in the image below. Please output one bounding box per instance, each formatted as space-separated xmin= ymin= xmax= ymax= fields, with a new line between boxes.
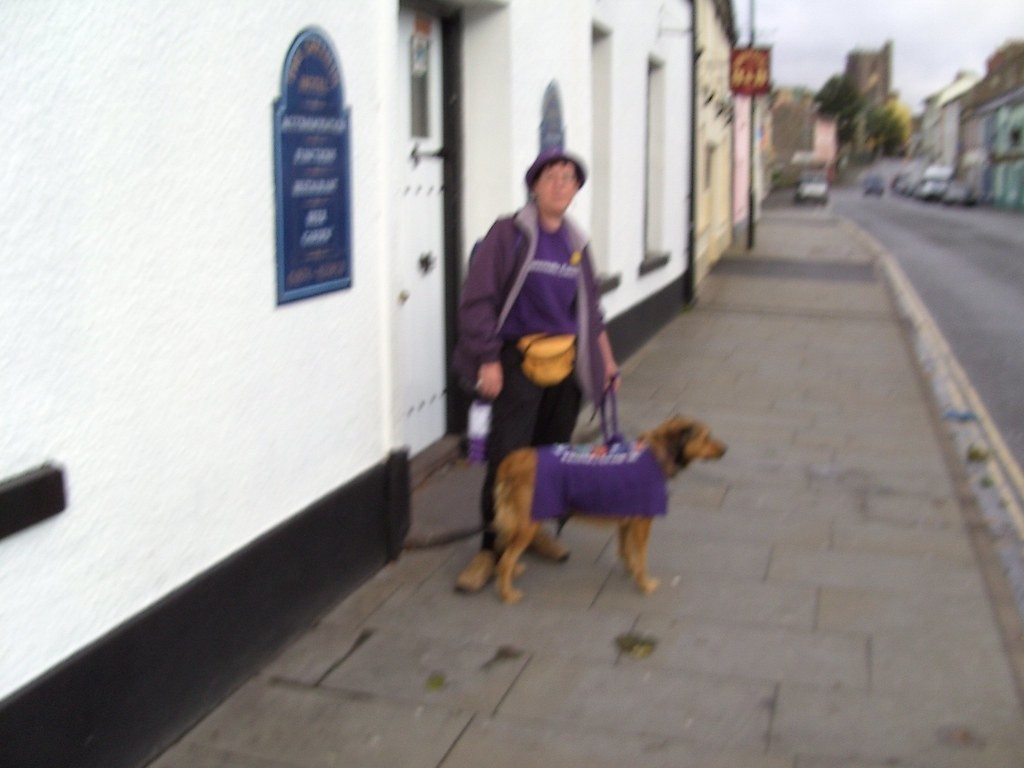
xmin=455 ymin=149 xmax=622 ymax=593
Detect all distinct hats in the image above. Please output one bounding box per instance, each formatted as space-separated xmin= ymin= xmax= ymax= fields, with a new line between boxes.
xmin=526 ymin=147 xmax=588 ymax=191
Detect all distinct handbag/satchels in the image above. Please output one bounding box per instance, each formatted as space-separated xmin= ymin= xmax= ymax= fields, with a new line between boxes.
xmin=518 ymin=330 xmax=577 ymax=386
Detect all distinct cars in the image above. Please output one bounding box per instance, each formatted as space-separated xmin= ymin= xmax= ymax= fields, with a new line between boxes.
xmin=863 ymin=173 xmax=883 ymax=195
xmin=796 ymin=173 xmax=829 ymax=202
xmin=943 ymin=181 xmax=977 ymax=207
xmin=893 ymin=173 xmax=917 ymax=197
xmin=913 ymin=179 xmax=948 ymax=201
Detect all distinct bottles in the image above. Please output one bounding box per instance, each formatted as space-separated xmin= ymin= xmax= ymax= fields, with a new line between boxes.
xmin=465 ymin=398 xmax=493 ymax=465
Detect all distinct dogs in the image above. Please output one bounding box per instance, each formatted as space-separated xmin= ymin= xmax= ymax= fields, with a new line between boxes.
xmin=495 ymin=414 xmax=728 ymax=603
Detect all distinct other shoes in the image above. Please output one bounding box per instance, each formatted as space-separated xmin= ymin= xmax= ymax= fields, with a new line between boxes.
xmin=525 ymin=525 xmax=570 ymax=561
xmin=457 ymin=549 xmax=497 ymax=594
